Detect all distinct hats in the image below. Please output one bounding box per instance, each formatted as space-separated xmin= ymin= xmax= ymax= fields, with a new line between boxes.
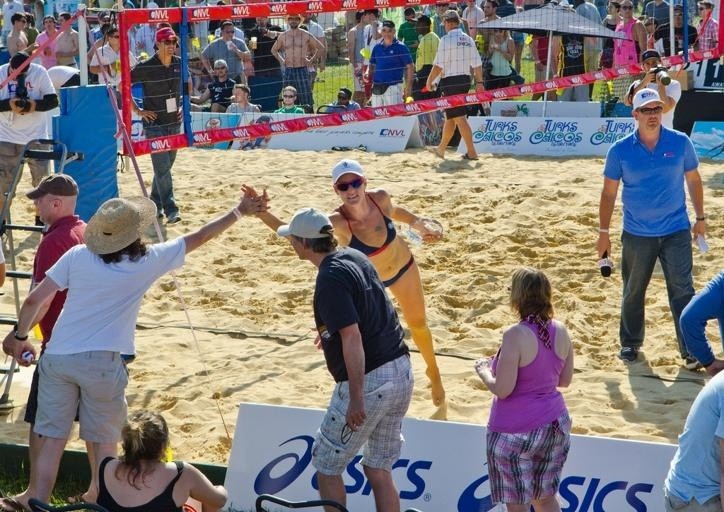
xmin=631 ymin=87 xmax=668 ymax=109
xmin=331 ymin=158 xmax=365 ymax=186
xmin=383 ymin=20 xmax=395 ymax=28
xmin=83 ymin=195 xmax=158 ymax=255
xmin=276 ymin=206 xmax=336 ymax=238
xmin=440 ymin=9 xmax=460 ymax=27
xmin=214 ymin=59 xmax=229 ymax=69
xmin=24 ymin=173 xmax=79 ymax=199
xmin=285 ymin=12 xmax=305 ymax=27
xmin=153 ymin=27 xmax=180 ymax=43
xmin=410 ymin=13 xmax=432 ymax=23
xmin=641 ymin=48 xmax=662 ymax=61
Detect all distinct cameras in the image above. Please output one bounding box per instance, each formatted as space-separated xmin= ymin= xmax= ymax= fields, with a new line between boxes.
xmin=15 ymin=74 xmax=30 ymax=107
xmin=650 ymin=64 xmax=671 ymax=85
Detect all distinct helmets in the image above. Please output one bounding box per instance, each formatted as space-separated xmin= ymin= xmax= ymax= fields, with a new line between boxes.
xmin=338 ymin=88 xmax=352 ymax=100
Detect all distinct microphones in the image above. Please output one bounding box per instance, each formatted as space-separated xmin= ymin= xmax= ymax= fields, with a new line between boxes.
xmin=598 ymin=250 xmax=614 ymax=276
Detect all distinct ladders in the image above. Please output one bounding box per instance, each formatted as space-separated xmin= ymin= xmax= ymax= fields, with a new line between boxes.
xmin=1 ymin=139 xmax=85 ymax=406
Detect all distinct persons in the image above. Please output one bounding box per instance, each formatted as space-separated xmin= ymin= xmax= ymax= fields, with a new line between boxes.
xmin=117 ymin=26 xmax=193 ymax=226
xmin=237 ymin=156 xmax=447 ymax=411
xmin=274 ymin=205 xmax=414 ymax=511
xmin=0 ymin=52 xmax=58 ymax=236
xmin=93 ymin=409 xmax=227 ymax=511
xmin=595 ymin=88 xmax=707 ymax=372
xmin=662 ymin=367 xmax=724 ymax=512
xmin=13 ymin=189 xmax=268 ymax=511
xmin=473 ymin=265 xmax=575 ymax=511
xmin=677 ymin=267 xmax=724 ymax=376
xmin=0 ymin=172 xmax=97 ymax=511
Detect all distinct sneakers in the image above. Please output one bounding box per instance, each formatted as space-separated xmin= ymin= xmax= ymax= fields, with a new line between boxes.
xmin=683 ymin=356 xmax=705 ymax=371
xmin=619 ymin=344 xmax=640 ymax=361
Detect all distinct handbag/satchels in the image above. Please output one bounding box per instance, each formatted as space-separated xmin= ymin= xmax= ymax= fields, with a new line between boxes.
xmin=371 ymin=82 xmax=390 ymax=95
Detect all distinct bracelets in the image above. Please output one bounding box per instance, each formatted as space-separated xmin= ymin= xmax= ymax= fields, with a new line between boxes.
xmin=695 ymin=216 xmax=704 ymax=221
xmin=231 ymin=205 xmax=242 ymax=222
xmin=598 ymin=228 xmax=609 ymax=233
xmin=13 ymin=324 xmax=27 ymax=341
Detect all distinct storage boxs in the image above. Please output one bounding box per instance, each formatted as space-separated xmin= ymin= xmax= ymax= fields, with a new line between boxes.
xmin=666 ymin=69 xmax=694 ymax=92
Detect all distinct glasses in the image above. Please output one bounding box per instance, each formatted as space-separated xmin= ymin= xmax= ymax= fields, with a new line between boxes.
xmin=215 ymin=65 xmax=226 ymax=71
xmin=641 ymin=105 xmax=661 ymax=111
xmin=223 ymin=30 xmax=235 ymax=33
xmin=283 ymin=93 xmax=294 ymax=100
xmin=622 ymin=4 xmax=634 ymax=12
xmin=160 ymin=39 xmax=177 ymax=45
xmin=336 ymin=179 xmax=363 ymax=191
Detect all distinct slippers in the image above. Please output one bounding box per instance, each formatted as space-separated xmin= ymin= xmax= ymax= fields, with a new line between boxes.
xmin=461 ymin=152 xmax=479 ymax=161
xmin=427 ymin=147 xmax=447 ymax=158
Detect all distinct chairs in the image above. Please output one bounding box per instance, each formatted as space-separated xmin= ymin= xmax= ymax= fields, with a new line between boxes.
xmin=253 ymin=103 xmax=347 ymax=117
